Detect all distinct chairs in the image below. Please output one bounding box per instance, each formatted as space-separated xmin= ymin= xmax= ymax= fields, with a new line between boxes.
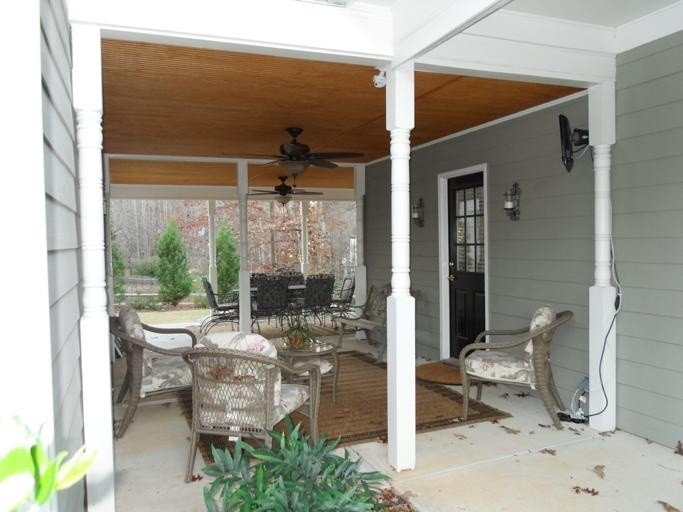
xmin=458 ymin=310 xmax=574 ymax=430
xmin=199 ymin=271 xmax=355 ymax=336
xmin=109 ymin=316 xmax=197 ymax=439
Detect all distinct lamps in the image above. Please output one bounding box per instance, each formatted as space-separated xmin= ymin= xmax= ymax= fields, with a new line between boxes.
xmin=277 ymin=160 xmax=310 ymax=187
xmin=411 ymin=198 xmax=425 ymax=228
xmin=502 ymin=182 xmax=522 ymax=221
xmin=274 ymin=196 xmax=293 ymax=207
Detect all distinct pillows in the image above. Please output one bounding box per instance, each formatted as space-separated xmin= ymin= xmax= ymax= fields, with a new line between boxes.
xmin=117 ymin=304 xmax=154 ymax=377
xmin=524 ymin=306 xmax=557 ymax=369
xmin=192 ymin=331 xmax=283 ymax=411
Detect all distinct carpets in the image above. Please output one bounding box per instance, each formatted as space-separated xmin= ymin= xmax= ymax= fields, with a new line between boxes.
xmin=416 ymin=360 xmax=491 ymax=386
xmin=176 ymin=349 xmax=514 ymax=467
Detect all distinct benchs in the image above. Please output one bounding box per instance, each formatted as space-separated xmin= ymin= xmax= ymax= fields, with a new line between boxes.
xmin=330 ymin=282 xmax=421 ymax=366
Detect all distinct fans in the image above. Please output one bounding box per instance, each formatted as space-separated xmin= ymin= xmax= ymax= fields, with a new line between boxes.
xmin=246 ymin=176 xmax=324 ymax=196
xmin=220 ymin=127 xmax=365 ymax=169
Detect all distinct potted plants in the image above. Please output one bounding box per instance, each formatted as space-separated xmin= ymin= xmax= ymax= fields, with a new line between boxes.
xmin=279 ymin=313 xmax=319 ymax=350
